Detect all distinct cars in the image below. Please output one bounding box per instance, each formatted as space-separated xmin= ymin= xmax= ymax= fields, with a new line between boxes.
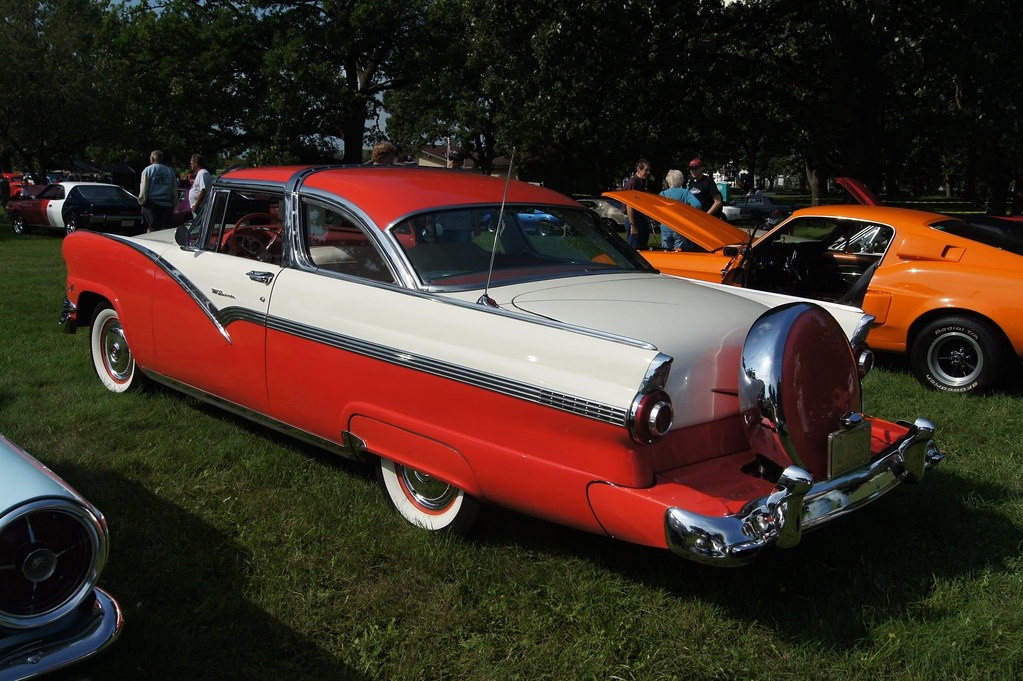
xmin=831 ymin=175 xmax=1022 ymax=242
xmin=58 ymin=159 xmax=944 ymax=573
xmin=0 ymin=432 xmax=126 ymax=681
xmin=475 ymin=205 xmax=579 ymax=239
xmin=592 ymin=183 xmax=1023 ymax=397
xmin=574 ymin=198 xmax=658 ymax=232
xmin=717 ymin=197 xmax=791 ymax=224
xmin=4 ymin=169 xmax=423 ymax=255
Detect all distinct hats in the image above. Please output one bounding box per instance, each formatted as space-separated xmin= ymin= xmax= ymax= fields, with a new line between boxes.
xmin=687 ymin=159 xmax=702 ymax=169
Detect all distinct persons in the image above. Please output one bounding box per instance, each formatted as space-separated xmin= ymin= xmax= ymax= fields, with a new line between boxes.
xmin=657 ymin=168 xmax=700 ymax=252
xmin=187 ymin=152 xmax=211 ymax=225
xmin=682 ymin=158 xmax=723 ymax=250
xmin=135 ymin=149 xmax=177 ymax=233
xmin=446 ymin=149 xmax=465 ymax=168
xmin=622 ymin=158 xmax=653 ymax=249
xmin=370 ymin=142 xmax=396 ymax=166
xmin=0 ymin=166 xmax=115 ymax=219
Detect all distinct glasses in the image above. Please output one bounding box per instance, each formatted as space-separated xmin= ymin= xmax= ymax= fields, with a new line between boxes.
xmin=689 ymin=166 xmax=700 ymax=170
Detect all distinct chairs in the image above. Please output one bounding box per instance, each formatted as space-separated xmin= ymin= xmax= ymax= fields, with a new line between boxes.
xmin=305 ymin=216 xmax=489 ymax=272
xmin=813 ymin=257 xmax=881 ymax=305
